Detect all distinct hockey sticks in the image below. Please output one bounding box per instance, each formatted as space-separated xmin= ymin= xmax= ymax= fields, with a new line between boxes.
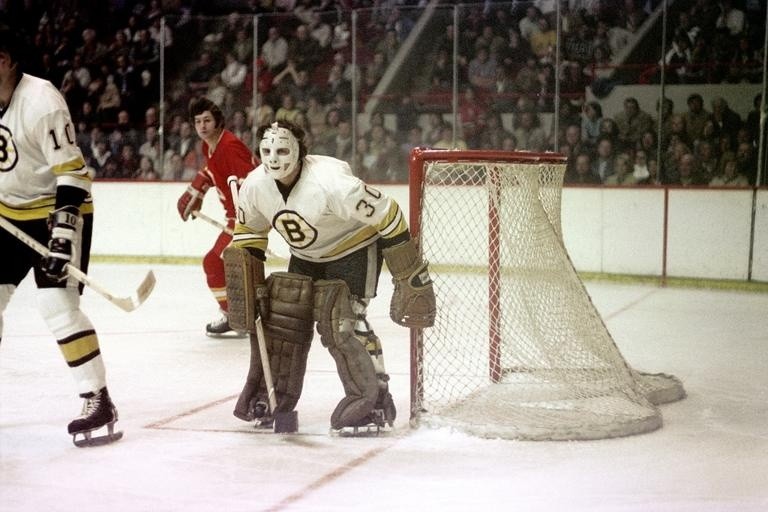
xmin=0 ymin=216 xmax=156 ymax=312
xmin=226 ymin=174 xmax=297 ymax=433
xmin=193 ymin=210 xmax=291 ymax=261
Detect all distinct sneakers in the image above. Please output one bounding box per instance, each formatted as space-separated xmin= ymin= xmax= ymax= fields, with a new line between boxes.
xmin=340 ymin=412 xmax=391 ymax=427
xmin=204 ymin=320 xmax=232 ymax=334
xmin=62 ymin=389 xmax=120 ymax=434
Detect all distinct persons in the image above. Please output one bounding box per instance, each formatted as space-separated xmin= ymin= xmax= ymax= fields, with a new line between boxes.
xmin=232 ymin=118 xmax=438 ymax=430
xmin=0 ymin=30 xmax=119 ymax=436
xmin=174 ymin=98 xmax=265 ymax=335
xmin=1 ymin=2 xmax=768 ymax=188
